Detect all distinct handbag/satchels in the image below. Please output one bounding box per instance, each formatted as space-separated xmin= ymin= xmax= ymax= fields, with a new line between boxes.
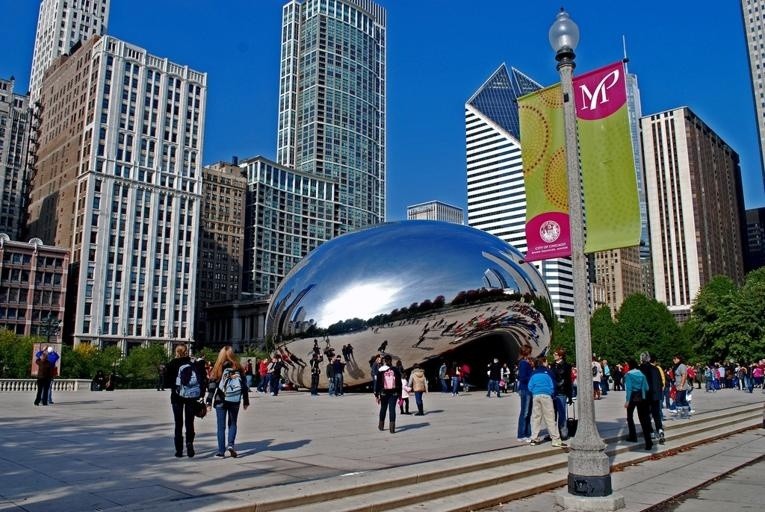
xmin=192 ymin=401 xmax=209 ymax=420
xmin=218 ymin=366 xmax=242 ymax=405
xmin=175 ymin=363 xmax=202 ymax=400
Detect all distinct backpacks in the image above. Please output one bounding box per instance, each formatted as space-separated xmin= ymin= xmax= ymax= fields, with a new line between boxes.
xmin=382 ymin=370 xmax=397 ymax=391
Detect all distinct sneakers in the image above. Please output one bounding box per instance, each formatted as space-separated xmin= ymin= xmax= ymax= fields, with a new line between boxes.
xmin=227 ymin=447 xmax=239 ymax=458
xmin=31 ymin=400 xmax=54 ymax=407
xmin=665 ymin=405 xmax=696 ymax=414
xmin=485 ymin=388 xmax=507 ymax=398
xmin=627 ymin=430 xmax=666 ymax=451
xmin=310 ymin=392 xmax=346 ymax=397
xmin=515 ymin=435 xmax=570 ymax=450
xmin=399 ymin=403 xmax=424 ymax=416
xmin=247 ymin=387 xmax=279 ymax=397
xmin=451 ymin=386 xmax=469 ymax=398
xmin=214 ymin=452 xmax=226 ymax=459
xmin=591 ymin=385 xmax=626 ymax=400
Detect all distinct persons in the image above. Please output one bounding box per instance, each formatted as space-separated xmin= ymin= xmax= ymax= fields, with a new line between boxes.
xmin=36 ymin=345 xmax=60 ymax=404
xmin=34 ymin=351 xmax=53 ymax=405
xmin=274 ymin=301 xmax=543 ymax=371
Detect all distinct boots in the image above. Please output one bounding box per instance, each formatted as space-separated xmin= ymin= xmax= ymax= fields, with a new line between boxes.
xmin=389 ymin=421 xmax=396 ymax=434
xmin=377 ymin=419 xmax=385 ymax=431
xmin=172 ymin=434 xmax=185 ymax=458
xmin=185 ymin=431 xmax=195 ymax=458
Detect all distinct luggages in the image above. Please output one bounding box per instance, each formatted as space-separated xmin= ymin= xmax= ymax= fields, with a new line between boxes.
xmin=565 ymin=401 xmax=579 ymax=438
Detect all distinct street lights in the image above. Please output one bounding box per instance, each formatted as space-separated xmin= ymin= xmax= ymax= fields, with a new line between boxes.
xmin=94 ymin=325 xmax=193 ymax=360
xmin=547 ymin=7 xmax=614 ymax=499
xmin=38 ymin=311 xmax=62 ymax=342
